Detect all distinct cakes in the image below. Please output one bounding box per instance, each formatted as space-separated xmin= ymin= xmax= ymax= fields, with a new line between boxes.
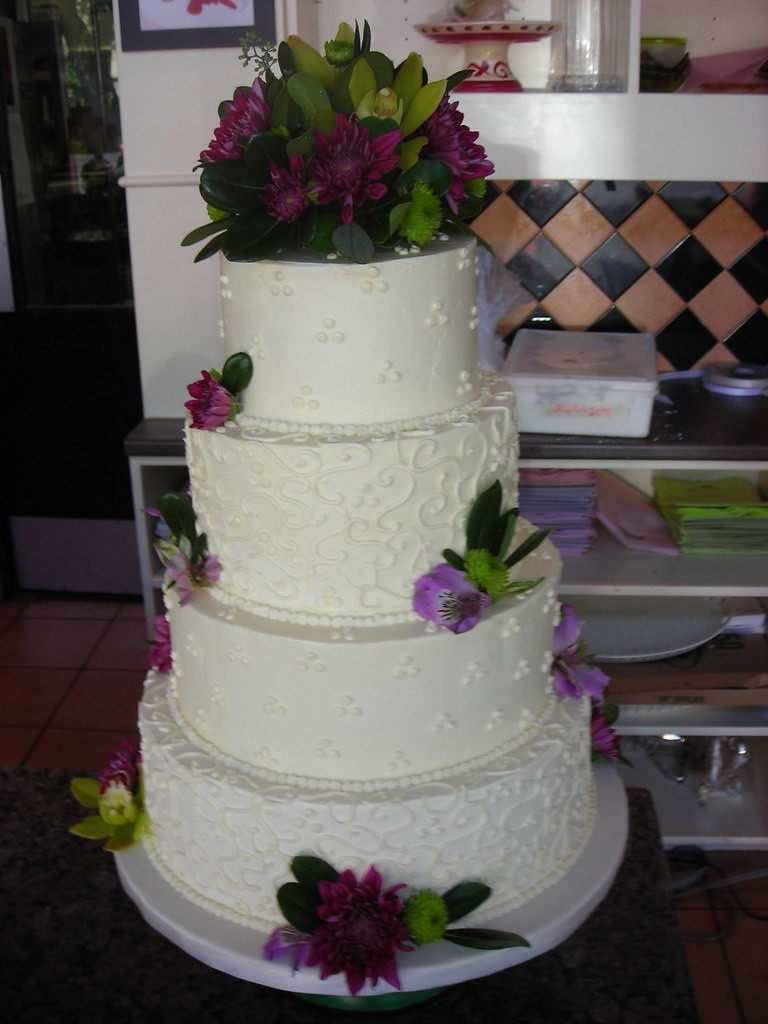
xmin=70 ymin=22 xmax=635 ymax=996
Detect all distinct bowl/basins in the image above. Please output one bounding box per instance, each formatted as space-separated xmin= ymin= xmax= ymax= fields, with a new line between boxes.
xmin=560 ymin=595 xmax=734 ymax=660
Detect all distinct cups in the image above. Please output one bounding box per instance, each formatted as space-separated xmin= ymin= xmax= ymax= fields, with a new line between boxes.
xmin=640 ymin=37 xmax=692 ymax=92
xmin=547 ymin=0 xmax=631 ymax=93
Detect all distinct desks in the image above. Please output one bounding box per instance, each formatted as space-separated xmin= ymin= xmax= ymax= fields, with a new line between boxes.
xmin=0 ymin=766 xmax=699 ymax=1024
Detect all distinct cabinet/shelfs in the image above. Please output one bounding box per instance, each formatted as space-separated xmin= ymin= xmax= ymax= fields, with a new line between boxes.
xmin=125 ymin=388 xmax=768 ymax=869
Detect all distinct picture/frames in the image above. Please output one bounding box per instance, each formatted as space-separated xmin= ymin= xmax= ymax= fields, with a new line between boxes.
xmin=117 ymin=0 xmax=277 ymax=52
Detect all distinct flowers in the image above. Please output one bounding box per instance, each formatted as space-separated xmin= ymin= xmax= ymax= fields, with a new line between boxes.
xmin=261 ymin=857 xmax=532 ymax=996
xmin=184 ymin=352 xmax=253 ymax=430
xmin=66 ymin=739 xmax=151 ymax=852
xmin=413 ymin=479 xmax=552 ymax=635
xmin=143 ymin=614 xmax=172 ymax=673
xmin=180 ymin=19 xmax=495 ymax=263
xmin=590 ymin=687 xmax=635 ymax=769
xmin=551 ymin=603 xmax=612 ymax=700
xmin=140 ymin=490 xmax=221 ymax=606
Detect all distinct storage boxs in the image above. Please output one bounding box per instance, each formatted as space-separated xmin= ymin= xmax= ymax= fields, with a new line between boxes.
xmin=606 ymin=632 xmax=768 ymax=706
xmin=504 ymin=330 xmax=658 ymax=438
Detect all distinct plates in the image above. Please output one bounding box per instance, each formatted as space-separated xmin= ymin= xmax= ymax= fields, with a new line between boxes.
xmin=700 ymin=83 xmax=768 ymax=93
